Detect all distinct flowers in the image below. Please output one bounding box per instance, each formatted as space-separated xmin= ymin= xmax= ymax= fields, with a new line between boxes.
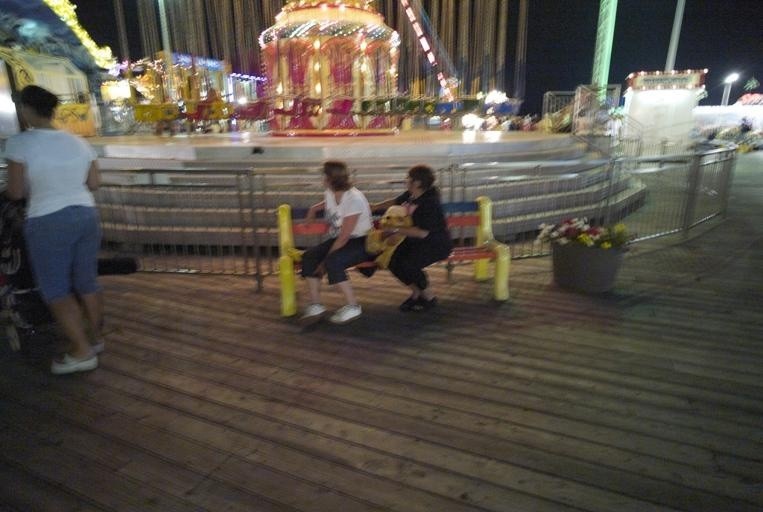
xmin=533 ymin=217 xmax=637 ymax=251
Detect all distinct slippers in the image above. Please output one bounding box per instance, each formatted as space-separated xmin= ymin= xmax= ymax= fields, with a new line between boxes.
xmin=401 ymin=296 xmax=437 ymax=312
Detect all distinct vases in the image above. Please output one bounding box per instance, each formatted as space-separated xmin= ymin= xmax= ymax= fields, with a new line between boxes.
xmin=552 ymin=245 xmax=626 ymax=294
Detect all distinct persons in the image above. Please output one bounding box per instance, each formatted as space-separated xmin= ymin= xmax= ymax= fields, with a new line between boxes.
xmin=739 ymin=116 xmax=752 ymax=133
xmin=153 ymin=116 xmax=257 ymax=136
xmin=398 ymin=110 xmax=555 ymax=132
xmin=1 ymin=85 xmax=107 ymax=378
xmin=297 ymin=161 xmax=376 ymax=328
xmin=369 ymin=164 xmax=455 ymax=314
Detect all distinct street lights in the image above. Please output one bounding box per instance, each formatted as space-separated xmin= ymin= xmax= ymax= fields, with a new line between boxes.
xmin=720 ymin=72 xmax=740 ymax=105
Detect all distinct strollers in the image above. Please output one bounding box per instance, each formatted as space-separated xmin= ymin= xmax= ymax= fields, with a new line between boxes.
xmin=2 ymin=195 xmax=56 ymax=352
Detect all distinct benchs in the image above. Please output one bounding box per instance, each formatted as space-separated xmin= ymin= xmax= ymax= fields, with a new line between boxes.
xmin=280 ymin=195 xmax=511 ymax=317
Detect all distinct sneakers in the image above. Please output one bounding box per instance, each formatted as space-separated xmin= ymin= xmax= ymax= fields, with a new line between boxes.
xmin=300 ymin=305 xmax=328 ymax=326
xmin=51 ymin=333 xmax=105 ymax=375
xmin=330 ymin=305 xmax=362 ymax=323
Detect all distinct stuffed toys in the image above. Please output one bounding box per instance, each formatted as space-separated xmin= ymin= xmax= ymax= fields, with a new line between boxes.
xmin=365 ymin=200 xmax=418 ymax=271
